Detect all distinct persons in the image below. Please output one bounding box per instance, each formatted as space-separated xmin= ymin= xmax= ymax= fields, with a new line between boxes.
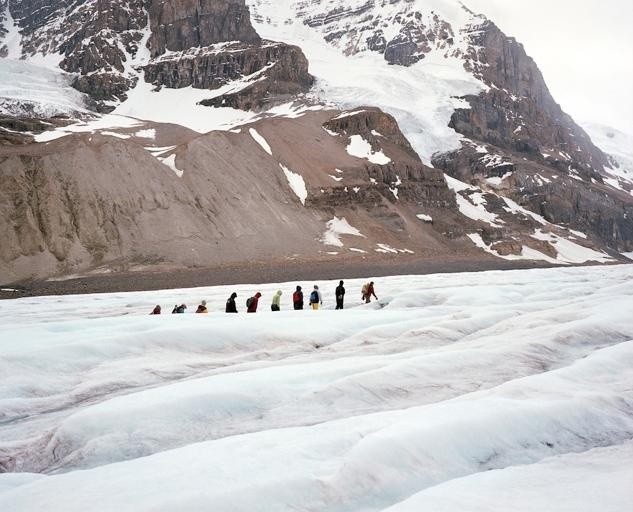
xmin=172 ymin=303 xmax=186 ymax=313
xmin=309 ymin=285 xmax=322 ymax=310
xmin=335 ymin=280 xmax=345 ymax=310
xmin=196 ymin=300 xmax=207 ymax=313
xmin=226 ymin=292 xmax=239 ymax=313
xmin=245 ymin=292 xmax=261 ymax=312
xmin=150 ymin=304 xmax=161 ymax=314
xmin=362 ymin=282 xmax=378 ymax=303
xmin=271 ymin=289 xmax=283 ymax=311
xmin=293 ymin=286 xmax=303 ymax=310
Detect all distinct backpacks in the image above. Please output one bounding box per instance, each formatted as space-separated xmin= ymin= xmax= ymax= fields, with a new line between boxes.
xmin=293 ymin=292 xmax=300 ymax=302
xmin=247 ymin=297 xmax=253 ymax=307
xmin=362 ymin=283 xmax=369 ymax=294
xmin=311 ymin=291 xmax=318 ymax=303
xmin=172 ymin=307 xmax=184 ymax=313
